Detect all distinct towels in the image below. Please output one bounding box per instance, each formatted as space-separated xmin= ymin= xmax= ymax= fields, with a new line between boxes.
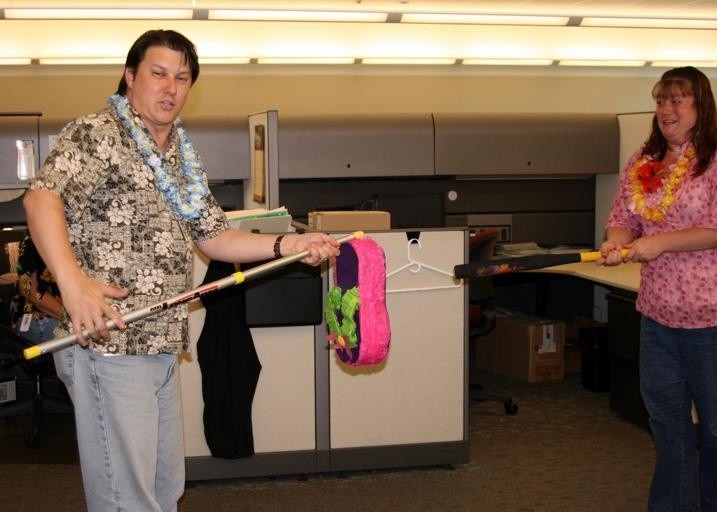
xmin=194 ymin=260 xmax=262 ymax=460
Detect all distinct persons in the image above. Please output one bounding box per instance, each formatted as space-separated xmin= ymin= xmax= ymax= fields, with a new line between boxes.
xmin=594 ymin=65 xmax=715 ymax=509
xmin=13 ymin=230 xmax=67 ymax=397
xmin=19 ymin=28 xmax=344 ymax=512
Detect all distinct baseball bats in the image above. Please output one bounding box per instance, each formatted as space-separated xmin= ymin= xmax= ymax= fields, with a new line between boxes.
xmin=455 ymin=249 xmax=632 ymax=280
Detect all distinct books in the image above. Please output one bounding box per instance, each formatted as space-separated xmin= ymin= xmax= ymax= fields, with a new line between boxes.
xmin=224 ymin=204 xmax=291 ymax=233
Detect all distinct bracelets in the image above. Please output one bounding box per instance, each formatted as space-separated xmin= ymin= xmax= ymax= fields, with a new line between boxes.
xmin=274 ymin=234 xmax=284 ymax=261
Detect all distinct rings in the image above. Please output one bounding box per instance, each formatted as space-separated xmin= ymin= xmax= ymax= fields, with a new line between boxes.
xmin=319 ymin=257 xmax=327 ymax=263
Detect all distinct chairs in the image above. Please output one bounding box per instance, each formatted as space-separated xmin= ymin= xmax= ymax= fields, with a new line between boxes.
xmin=0 ymin=324 xmax=75 ymax=448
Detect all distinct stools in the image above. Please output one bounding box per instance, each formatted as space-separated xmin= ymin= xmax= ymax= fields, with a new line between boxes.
xmin=469 ymin=300 xmax=518 ymax=414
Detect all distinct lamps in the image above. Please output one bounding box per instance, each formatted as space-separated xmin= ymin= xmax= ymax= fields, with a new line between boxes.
xmin=0 ymin=9 xmax=716 ymax=29
xmin=2 ymin=51 xmax=716 ymax=68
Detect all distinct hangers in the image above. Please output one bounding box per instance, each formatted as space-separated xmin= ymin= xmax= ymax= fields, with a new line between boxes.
xmin=385 ymin=239 xmax=464 ymax=293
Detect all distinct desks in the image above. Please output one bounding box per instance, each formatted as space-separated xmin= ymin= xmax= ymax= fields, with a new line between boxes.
xmin=471 ymin=240 xmax=641 ymax=299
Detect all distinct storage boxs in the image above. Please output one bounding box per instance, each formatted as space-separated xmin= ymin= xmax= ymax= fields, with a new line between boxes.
xmin=308 ymin=211 xmax=391 ymax=231
xmin=475 ymin=307 xmax=566 ymax=382
